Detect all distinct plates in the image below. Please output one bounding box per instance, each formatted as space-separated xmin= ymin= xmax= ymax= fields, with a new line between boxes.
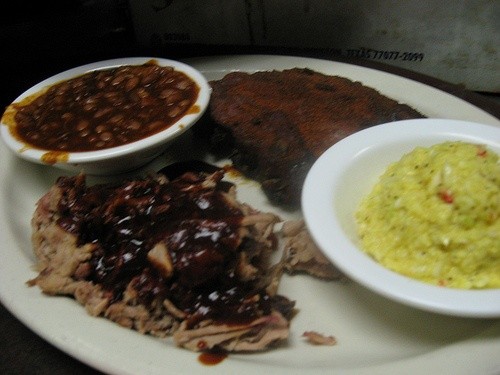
xmin=0 ymin=54 xmax=500 ymax=374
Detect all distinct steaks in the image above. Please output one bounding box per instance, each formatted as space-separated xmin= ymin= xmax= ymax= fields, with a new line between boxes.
xmin=204 ymin=66 xmax=430 ymax=212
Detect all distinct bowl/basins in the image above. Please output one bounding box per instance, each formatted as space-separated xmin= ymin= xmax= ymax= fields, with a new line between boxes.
xmin=301 ymin=118 xmax=500 ymax=317
xmin=0 ymin=57 xmax=211 ymax=175
